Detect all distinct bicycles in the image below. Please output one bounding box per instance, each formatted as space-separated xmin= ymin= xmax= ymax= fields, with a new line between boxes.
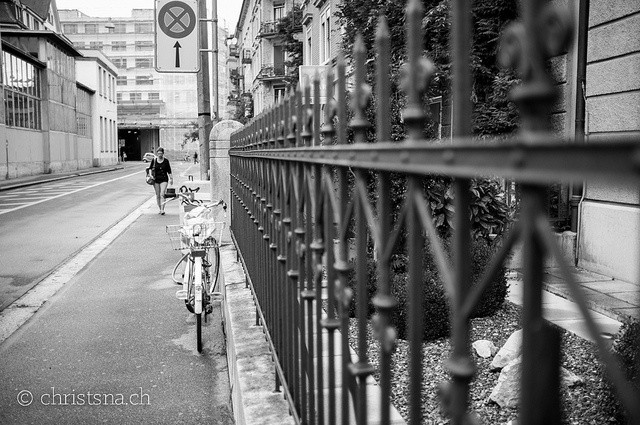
xmin=161 ymin=186 xmax=227 ymax=354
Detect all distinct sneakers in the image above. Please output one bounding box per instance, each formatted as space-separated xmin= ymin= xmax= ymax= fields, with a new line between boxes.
xmin=158 ymin=210 xmax=161 ymax=214
xmin=161 ymin=211 xmax=165 ymax=215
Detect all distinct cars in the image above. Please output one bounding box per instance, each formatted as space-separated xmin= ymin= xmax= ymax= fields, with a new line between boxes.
xmin=143 ymin=152 xmax=159 ymax=163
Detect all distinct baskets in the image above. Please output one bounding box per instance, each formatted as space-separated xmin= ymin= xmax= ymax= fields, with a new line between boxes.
xmin=166 ymin=221 xmax=227 ymax=251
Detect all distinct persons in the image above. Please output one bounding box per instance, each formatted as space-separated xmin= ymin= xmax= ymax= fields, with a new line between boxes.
xmin=146 ymin=146 xmax=175 ymax=215
xmin=120 ymin=150 xmax=128 ymax=163
xmin=191 ymin=150 xmax=199 ymax=163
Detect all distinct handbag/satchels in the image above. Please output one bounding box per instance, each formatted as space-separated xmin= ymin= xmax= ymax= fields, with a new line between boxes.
xmin=146 ymin=173 xmax=155 ymax=185
